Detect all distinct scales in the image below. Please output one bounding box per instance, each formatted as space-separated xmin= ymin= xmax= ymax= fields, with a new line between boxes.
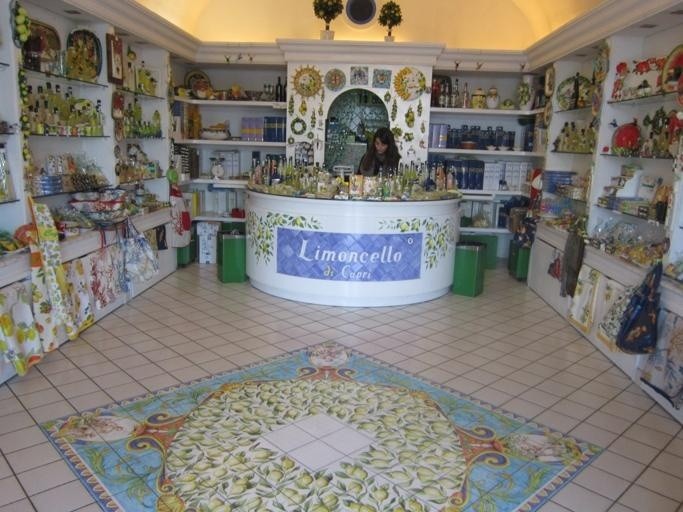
xmin=209 ymin=158 xmax=224 ymax=180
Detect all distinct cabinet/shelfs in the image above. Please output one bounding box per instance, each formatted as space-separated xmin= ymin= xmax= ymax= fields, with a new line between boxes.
xmin=529 ymin=4 xmax=682 ymax=424
xmin=173 ymin=53 xmax=288 ymax=265
xmin=430 ymin=61 xmax=538 ymax=233
xmin=1 ymin=1 xmax=174 ymax=383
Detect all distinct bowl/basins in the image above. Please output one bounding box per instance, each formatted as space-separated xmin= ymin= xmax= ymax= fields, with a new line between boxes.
xmin=513 ymin=146 xmax=522 ymax=151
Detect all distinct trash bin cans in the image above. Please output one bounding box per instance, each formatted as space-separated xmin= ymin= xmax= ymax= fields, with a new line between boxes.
xmin=453 ymin=242 xmax=485 ymax=297
xmin=509 ymin=246 xmax=530 ymax=280
xmin=217 ymin=231 xmax=246 ymax=283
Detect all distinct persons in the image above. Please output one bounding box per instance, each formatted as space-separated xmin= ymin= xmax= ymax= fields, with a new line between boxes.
xmin=358 ymin=128 xmax=402 ymax=176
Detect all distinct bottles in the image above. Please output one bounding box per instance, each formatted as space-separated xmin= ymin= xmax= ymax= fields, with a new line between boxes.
xmin=379 ymin=161 xmax=455 ymax=199
xmin=27 ymin=60 xmax=163 ymax=137
xmin=0 ymin=142 xmax=8 ymax=200
xmin=275 ymin=76 xmax=287 ymax=101
xmin=432 ymin=76 xmax=515 ymax=150
xmin=250 ymin=155 xmax=327 ymax=193
